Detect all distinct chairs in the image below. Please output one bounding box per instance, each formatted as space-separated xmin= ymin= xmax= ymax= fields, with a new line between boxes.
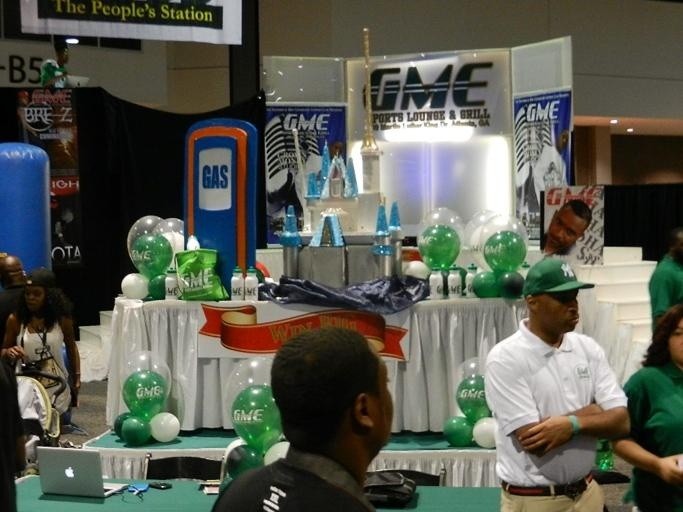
xmin=144 ymin=453 xmax=226 ymax=481
xmin=371 ymin=468 xmax=446 ymax=488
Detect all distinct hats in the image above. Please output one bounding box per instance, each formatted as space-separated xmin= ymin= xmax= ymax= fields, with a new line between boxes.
xmin=24 ymin=269 xmax=58 ymax=289
xmin=523 ymin=258 xmax=596 ymax=300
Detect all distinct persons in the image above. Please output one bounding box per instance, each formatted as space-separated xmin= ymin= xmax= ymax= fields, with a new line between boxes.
xmin=483 ymin=257 xmax=630 ymax=512
xmin=0 ymin=266 xmax=80 ymax=448
xmin=0 ymin=253 xmax=28 ymax=345
xmin=0 ymin=361 xmax=26 ymax=512
xmin=611 ymin=304 xmax=683 ymax=512
xmin=649 ymin=227 xmax=683 ymax=334
xmin=543 ymin=200 xmax=592 ymax=255
xmin=210 ymin=326 xmax=394 ymax=512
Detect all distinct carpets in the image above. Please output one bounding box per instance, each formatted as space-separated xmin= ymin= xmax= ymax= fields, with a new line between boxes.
xmin=59 ymin=379 xmax=110 ymax=444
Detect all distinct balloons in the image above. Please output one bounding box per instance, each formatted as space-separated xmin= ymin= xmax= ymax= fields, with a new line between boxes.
xmin=220 ymin=476 xmax=233 ymax=494
xmin=455 ymin=376 xmax=492 ymax=424
xmin=232 ymin=384 xmax=282 ymax=454
xmin=148 ymin=275 xmax=166 ymax=300
xmin=227 ymin=445 xmax=262 ymax=479
xmin=456 ymin=357 xmax=486 ymax=391
xmin=416 ymin=207 xmax=464 ymax=270
xmin=471 ymin=272 xmax=497 ymax=298
xmin=132 ymin=233 xmax=173 ymax=279
xmin=149 ymin=412 xmax=179 ymax=442
xmin=472 ymin=417 xmax=496 ymax=448
xmin=466 ymin=210 xmax=495 ymax=270
xmin=115 ymin=413 xmax=135 ymax=441
xmin=121 ymin=417 xmax=151 ymax=446
xmin=479 ymin=216 xmax=528 ymax=274
xmin=419 ymin=224 xmax=460 ymax=270
xmin=123 ymin=369 xmax=166 ymax=422
xmin=226 ymin=355 xmax=282 ymax=454
xmin=264 ymin=441 xmax=290 ymax=465
xmin=121 ymin=273 xmax=148 ymax=300
xmin=163 ymin=233 xmax=184 ymax=271
xmin=127 ymin=215 xmax=175 ymax=279
xmin=496 ymin=271 xmax=524 ymax=299
xmin=121 ymin=350 xmax=171 ymax=402
xmin=484 ymin=230 xmax=527 ymax=274
xmin=442 ymin=416 xmax=475 ymax=447
xmin=154 ymin=218 xmax=184 ymax=234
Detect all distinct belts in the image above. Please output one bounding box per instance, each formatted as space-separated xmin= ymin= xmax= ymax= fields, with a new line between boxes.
xmin=501 ymin=475 xmax=598 ymax=497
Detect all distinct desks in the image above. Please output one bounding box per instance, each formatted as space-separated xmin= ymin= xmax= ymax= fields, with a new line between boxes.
xmin=83 ymin=429 xmax=499 ymax=486
xmin=106 ymin=289 xmax=528 ymax=432
xmin=15 ymin=475 xmax=501 ymax=512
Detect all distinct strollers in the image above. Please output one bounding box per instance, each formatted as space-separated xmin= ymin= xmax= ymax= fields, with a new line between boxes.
xmin=15 ymin=371 xmax=74 ymax=478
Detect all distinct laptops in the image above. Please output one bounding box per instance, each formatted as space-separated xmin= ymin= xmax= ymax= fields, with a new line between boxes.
xmin=36 ymin=445 xmax=129 ymax=498
